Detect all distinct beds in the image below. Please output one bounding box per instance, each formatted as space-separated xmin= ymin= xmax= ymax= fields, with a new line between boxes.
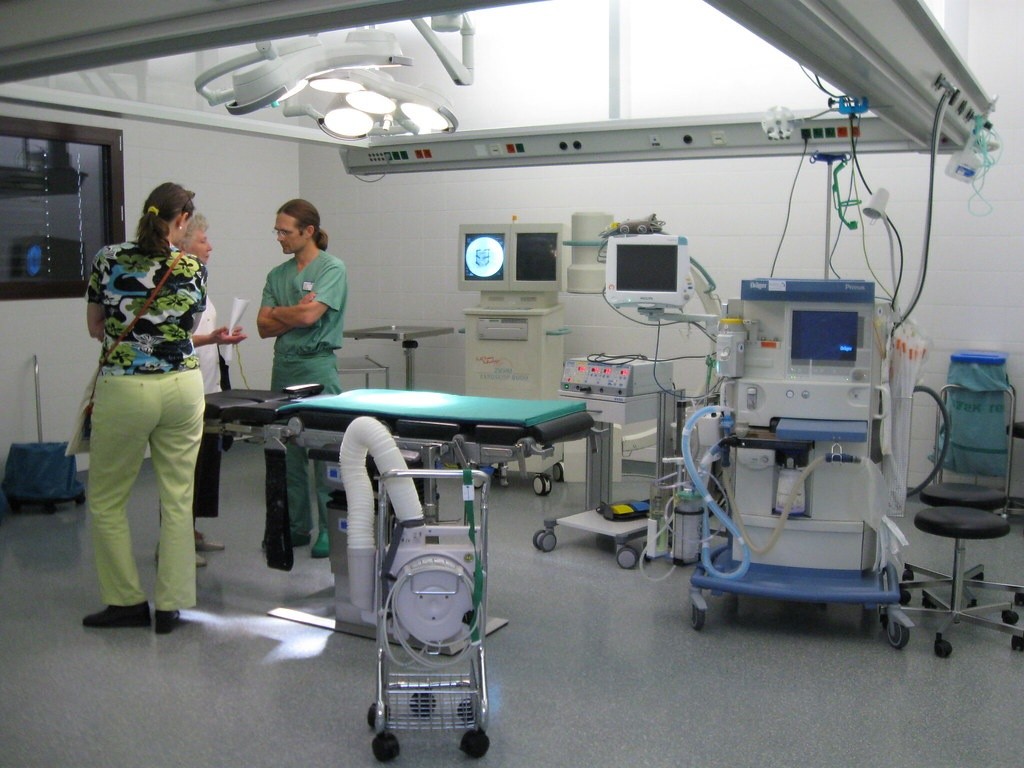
xmin=204 ymin=387 xmax=596 ymax=653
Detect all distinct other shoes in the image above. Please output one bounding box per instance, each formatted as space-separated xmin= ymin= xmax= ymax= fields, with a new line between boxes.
xmin=82 ymin=600 xmax=151 ymax=627
xmin=261 ymin=533 xmax=313 ymax=548
xmin=311 ymin=528 xmax=330 ymax=557
xmin=195 ymin=533 xmax=225 ymax=550
xmin=154 ymin=543 xmax=207 ymax=567
xmin=155 ymin=610 xmax=180 ymax=634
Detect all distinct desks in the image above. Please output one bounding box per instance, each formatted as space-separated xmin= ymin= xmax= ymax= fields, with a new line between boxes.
xmin=335 ymin=355 xmax=390 ymax=388
xmin=334 ymin=325 xmax=455 ymax=390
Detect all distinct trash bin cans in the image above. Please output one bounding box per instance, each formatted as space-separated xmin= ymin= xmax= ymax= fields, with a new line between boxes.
xmin=325 ymin=488 xmax=396 ymax=604
xmin=309 ymin=443 xmax=376 ymax=493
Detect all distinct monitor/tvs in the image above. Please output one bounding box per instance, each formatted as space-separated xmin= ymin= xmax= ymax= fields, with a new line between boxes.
xmin=603 ymin=234 xmax=689 ymax=307
xmin=785 ymin=305 xmax=861 ymax=368
xmin=509 ymin=223 xmax=563 ymax=292
xmin=457 ymin=224 xmax=508 ymax=291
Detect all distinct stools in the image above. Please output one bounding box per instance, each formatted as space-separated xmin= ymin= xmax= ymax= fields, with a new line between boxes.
xmin=877 ymin=507 xmax=1024 ymax=660
xmin=899 ymin=482 xmax=1024 ymax=607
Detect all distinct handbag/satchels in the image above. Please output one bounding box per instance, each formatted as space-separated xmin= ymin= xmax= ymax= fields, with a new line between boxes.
xmin=64 ymin=367 xmax=101 ymax=456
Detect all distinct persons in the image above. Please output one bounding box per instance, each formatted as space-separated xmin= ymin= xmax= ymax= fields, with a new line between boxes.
xmin=154 ymin=213 xmax=248 ymax=567
xmin=82 ymin=182 xmax=208 ymax=634
xmin=257 ymin=199 xmax=348 ymax=558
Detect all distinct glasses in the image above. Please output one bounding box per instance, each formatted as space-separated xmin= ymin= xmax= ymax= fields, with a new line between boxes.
xmin=182 ymin=190 xmax=196 ymax=210
xmin=271 ymin=229 xmax=287 ymax=238
